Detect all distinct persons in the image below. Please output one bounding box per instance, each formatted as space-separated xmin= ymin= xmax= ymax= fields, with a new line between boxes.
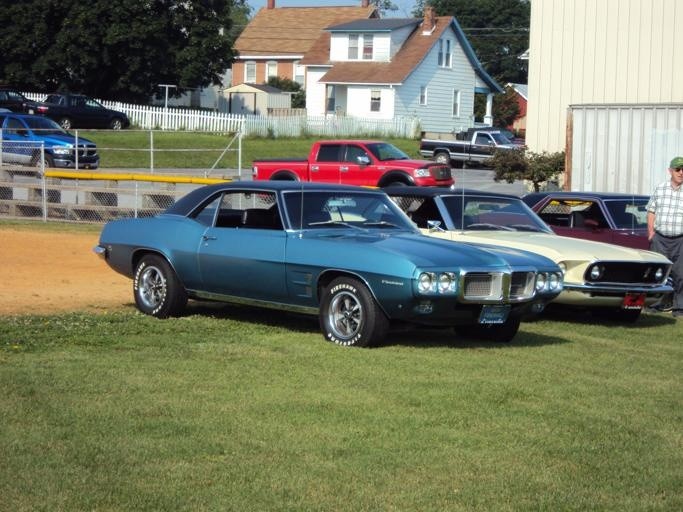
xmin=644 ymin=156 xmax=683 ymax=318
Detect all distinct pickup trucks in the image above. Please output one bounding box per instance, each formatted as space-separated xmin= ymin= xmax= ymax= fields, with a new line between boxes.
xmin=250 ymin=139 xmax=456 ymax=189
xmin=418 ymin=127 xmax=525 ymax=170
xmin=1 ymin=88 xmax=130 ymax=167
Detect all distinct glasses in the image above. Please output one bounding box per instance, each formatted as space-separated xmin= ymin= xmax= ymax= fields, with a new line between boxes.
xmin=670 ymin=168 xmax=683 ymax=172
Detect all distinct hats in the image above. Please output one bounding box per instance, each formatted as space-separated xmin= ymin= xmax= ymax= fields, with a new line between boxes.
xmin=670 ymin=157 xmax=683 ymax=167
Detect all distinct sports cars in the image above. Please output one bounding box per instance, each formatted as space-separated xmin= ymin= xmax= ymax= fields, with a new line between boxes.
xmin=91 ymin=179 xmax=563 ymax=349
xmin=471 ymin=191 xmax=653 ymax=255
xmin=331 ymin=184 xmax=675 ymax=325
